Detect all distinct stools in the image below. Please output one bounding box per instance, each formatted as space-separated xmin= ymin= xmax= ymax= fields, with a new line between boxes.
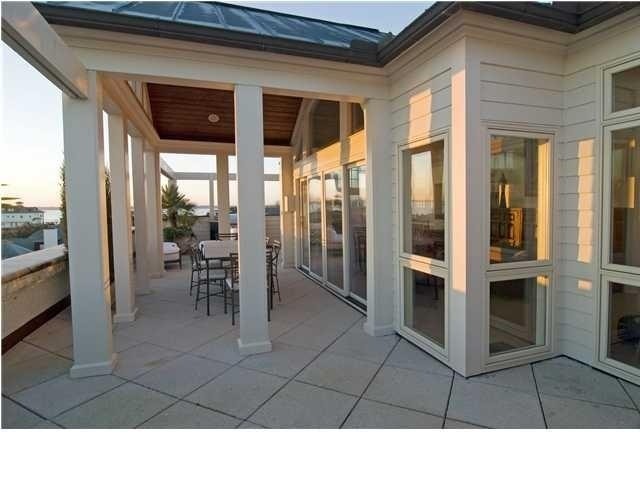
xmin=164 ymin=242 xmax=182 ymax=271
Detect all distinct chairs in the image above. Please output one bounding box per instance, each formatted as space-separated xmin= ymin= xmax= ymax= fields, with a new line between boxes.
xmin=188 ymin=230 xmax=281 ymax=325
